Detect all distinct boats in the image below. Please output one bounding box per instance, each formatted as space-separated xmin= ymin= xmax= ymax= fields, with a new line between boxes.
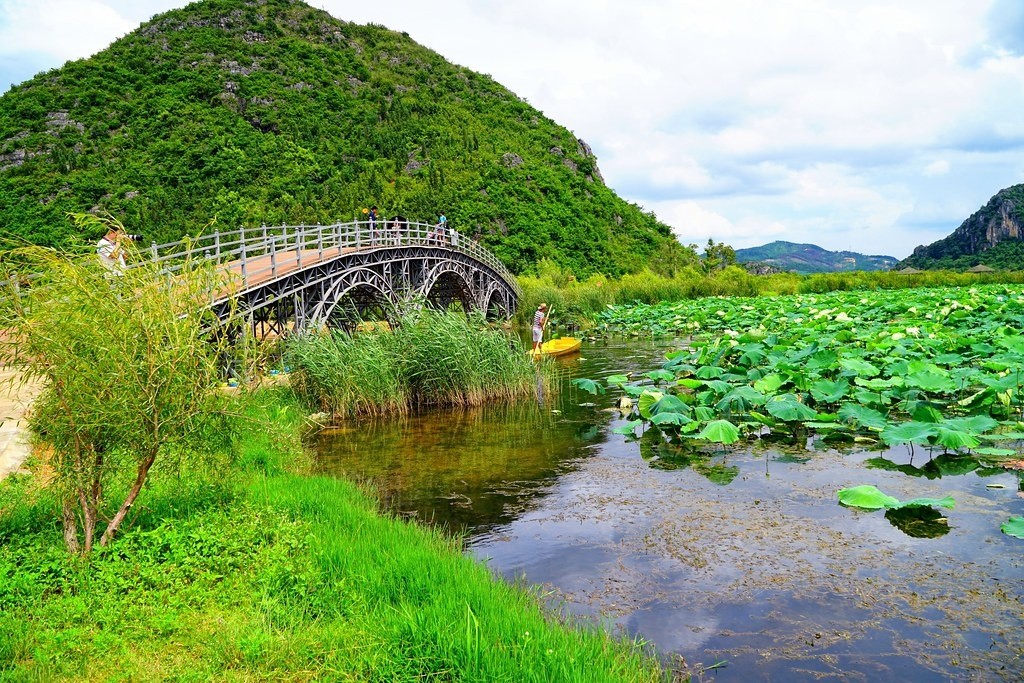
xmin=525 ymin=337 xmax=583 ymax=360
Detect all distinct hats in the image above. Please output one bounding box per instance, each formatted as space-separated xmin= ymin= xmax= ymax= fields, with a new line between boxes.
xmin=538 ymin=303 xmax=546 ymax=309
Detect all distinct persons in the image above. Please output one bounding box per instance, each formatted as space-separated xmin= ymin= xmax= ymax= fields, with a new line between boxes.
xmin=392 ymin=217 xmax=402 ymax=246
xmin=531 ymin=301 xmax=549 ymax=354
xmin=423 ymin=211 xmax=447 ymax=245
xmin=367 ymin=204 xmax=380 ymax=246
xmin=98 ymin=225 xmax=132 ymax=304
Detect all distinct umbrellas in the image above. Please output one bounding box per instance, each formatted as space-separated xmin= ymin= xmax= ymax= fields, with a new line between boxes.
xmin=387 ymin=215 xmax=408 ymax=234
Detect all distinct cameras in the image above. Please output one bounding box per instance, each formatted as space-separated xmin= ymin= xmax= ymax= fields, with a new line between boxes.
xmin=123 ymin=233 xmax=143 ymax=242
